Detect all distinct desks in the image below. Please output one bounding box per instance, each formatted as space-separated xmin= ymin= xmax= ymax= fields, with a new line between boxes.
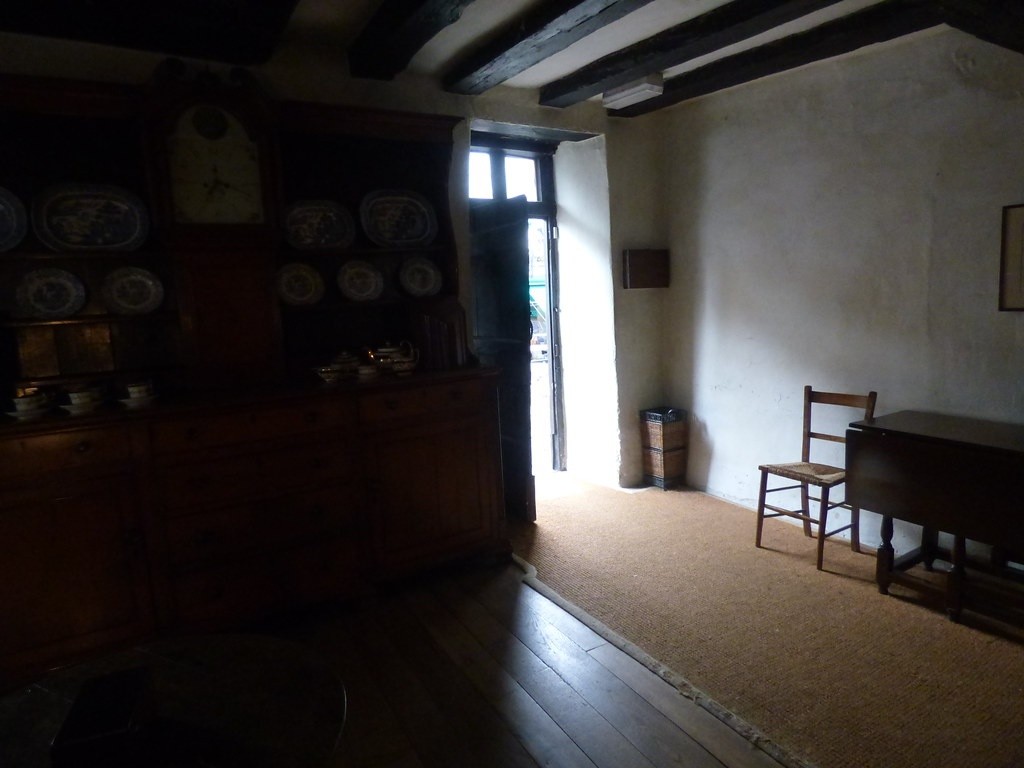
xmin=0 ymin=635 xmax=346 ymax=768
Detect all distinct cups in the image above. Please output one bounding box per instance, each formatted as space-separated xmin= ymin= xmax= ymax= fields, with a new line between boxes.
xmin=128 ymin=384 xmax=150 ymax=399
xmin=12 ymin=396 xmax=42 ymax=411
xmin=69 ymin=392 xmax=89 ymax=404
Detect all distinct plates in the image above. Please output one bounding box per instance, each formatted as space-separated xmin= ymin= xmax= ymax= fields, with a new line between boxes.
xmin=359 ymin=189 xmax=438 ymax=248
xmin=278 ymin=264 xmax=325 ymax=306
xmin=16 ymin=268 xmax=89 ymax=320
xmin=118 ymin=399 xmax=146 ymax=410
xmin=58 ymin=400 xmax=100 ymax=416
xmin=101 ymin=266 xmax=163 ymax=316
xmin=0 ymin=186 xmax=28 ymax=255
xmin=6 ymin=408 xmax=48 ymax=423
xmin=399 ymin=257 xmax=442 ymax=298
xmin=31 ymin=181 xmax=150 ymax=255
xmin=337 ymin=261 xmax=384 ymax=302
xmin=280 ymin=199 xmax=354 ymax=250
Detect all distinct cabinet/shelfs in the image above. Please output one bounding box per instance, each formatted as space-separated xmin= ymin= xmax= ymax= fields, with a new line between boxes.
xmin=845 ymin=410 xmax=1024 ymax=622
xmin=0 ymin=362 xmax=513 ymax=695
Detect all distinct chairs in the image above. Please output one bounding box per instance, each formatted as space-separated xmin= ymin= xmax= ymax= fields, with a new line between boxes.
xmin=755 ymin=385 xmax=877 ymax=570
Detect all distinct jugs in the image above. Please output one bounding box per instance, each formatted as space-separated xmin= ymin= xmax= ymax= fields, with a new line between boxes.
xmin=387 ymin=349 xmax=420 ymax=377
xmin=370 ymin=340 xmax=413 ymax=370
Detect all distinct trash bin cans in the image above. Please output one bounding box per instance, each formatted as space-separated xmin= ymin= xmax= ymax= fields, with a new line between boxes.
xmin=639 ymin=407 xmax=687 ymax=491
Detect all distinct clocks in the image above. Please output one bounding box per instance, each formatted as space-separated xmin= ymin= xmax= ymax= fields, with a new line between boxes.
xmin=158 ymin=95 xmax=269 ymax=231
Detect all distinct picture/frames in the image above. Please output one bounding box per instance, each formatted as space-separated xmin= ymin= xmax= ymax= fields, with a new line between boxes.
xmin=998 ymin=203 xmax=1024 ymax=312
